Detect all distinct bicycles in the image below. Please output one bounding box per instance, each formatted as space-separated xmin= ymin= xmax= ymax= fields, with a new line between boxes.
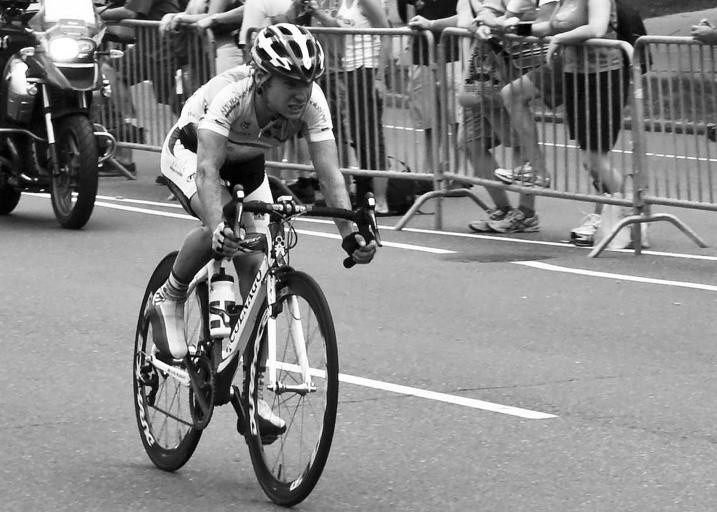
xmin=133 ymin=184 xmax=382 ymax=506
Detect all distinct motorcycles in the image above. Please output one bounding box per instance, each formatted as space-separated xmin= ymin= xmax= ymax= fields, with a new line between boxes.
xmin=1 ymin=1 xmax=137 ymax=228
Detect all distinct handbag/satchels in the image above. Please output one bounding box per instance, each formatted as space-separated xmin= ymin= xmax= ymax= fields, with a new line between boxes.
xmin=352 ymin=176 xmax=416 ymax=218
xmin=615 ymin=2 xmax=652 ymax=78
xmin=460 ymin=34 xmax=550 ymax=109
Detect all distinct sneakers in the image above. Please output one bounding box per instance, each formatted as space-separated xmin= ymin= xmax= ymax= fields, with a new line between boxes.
xmin=108 ymin=124 xmax=143 ymax=144
xmin=97 ymin=156 xmax=137 ymax=177
xmin=146 ymin=287 xmax=188 ymax=359
xmin=257 ymin=399 xmax=285 ymax=434
xmin=569 ymin=213 xmax=602 ymax=243
xmin=486 ymin=209 xmax=539 ymax=233
xmin=271 ymin=183 xmax=315 ymax=204
xmin=468 ymin=208 xmax=507 ymax=233
xmin=492 ymin=161 xmax=549 ymax=190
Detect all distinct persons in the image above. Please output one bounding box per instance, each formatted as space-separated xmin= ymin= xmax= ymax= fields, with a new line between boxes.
xmin=691 ymin=19 xmax=717 ymax=46
xmin=397 ymin=0 xmax=477 ymax=187
xmin=493 ymin=1 xmax=607 ymax=246
xmin=150 ymin=21 xmax=378 ymax=440
xmin=458 ymin=0 xmax=540 ymax=234
xmin=531 ymin=1 xmax=650 ymax=250
xmin=2 ymin=1 xmax=403 ymax=219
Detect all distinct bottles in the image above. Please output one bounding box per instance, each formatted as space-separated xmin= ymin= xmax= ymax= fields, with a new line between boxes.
xmin=209 ymin=266 xmax=238 ymax=339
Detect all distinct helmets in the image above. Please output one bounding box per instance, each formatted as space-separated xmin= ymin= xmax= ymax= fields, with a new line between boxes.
xmin=250 ymin=23 xmax=326 ymax=85
